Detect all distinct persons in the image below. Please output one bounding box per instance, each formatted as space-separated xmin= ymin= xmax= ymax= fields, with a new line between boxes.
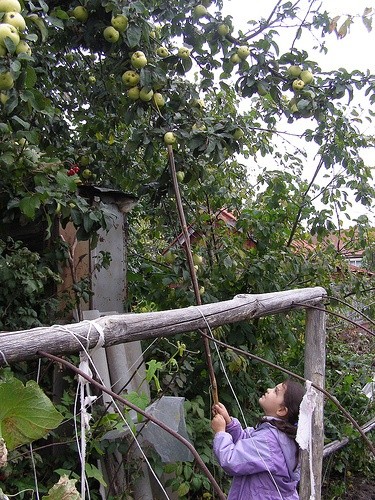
xmin=211 ymin=380 xmax=307 ymax=500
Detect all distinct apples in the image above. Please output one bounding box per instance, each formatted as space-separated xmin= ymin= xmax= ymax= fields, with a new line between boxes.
xmin=0 ymin=0 xmax=314 ymax=295
